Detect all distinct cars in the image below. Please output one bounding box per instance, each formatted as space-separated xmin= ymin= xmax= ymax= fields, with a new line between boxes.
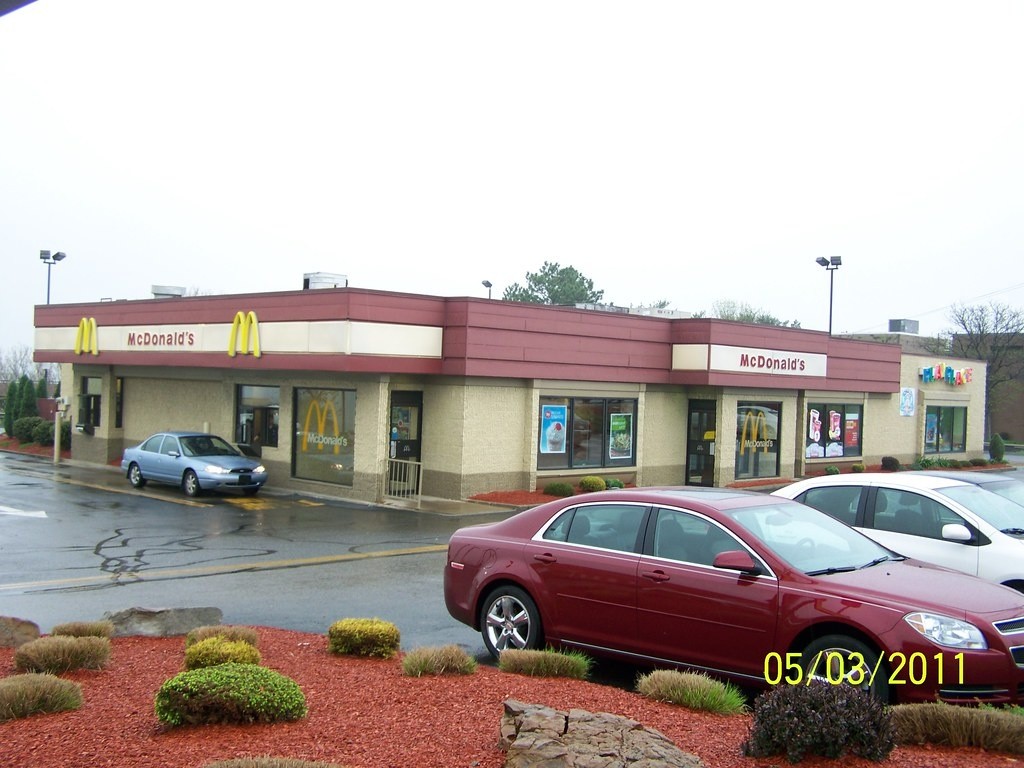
xmin=758 ymin=473 xmax=1024 ymax=593
xmin=565 ymin=409 xmax=592 ymax=447
xmin=737 ymin=407 xmax=778 ymax=441
xmin=442 ymin=485 xmax=1024 ymax=708
xmin=850 ymin=472 xmax=1024 ymax=523
xmin=295 ymin=431 xmax=354 ymax=485
xmin=121 ymin=432 xmax=267 ymax=499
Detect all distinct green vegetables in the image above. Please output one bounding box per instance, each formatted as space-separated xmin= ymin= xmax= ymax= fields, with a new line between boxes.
xmin=611 ymin=434 xmax=631 ymax=449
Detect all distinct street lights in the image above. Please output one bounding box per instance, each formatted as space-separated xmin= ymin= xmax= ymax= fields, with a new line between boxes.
xmin=816 ymin=256 xmax=842 ymax=336
xmin=482 ymin=280 xmax=493 ymax=298
xmin=40 ymin=249 xmax=66 ymax=381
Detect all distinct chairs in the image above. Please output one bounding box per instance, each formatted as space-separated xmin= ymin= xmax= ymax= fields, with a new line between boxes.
xmin=875 ymin=492 xmax=896 ymax=530
xmin=560 ymin=515 xmax=595 ymax=545
xmin=658 ymin=519 xmax=687 ymax=561
xmin=618 ymin=512 xmax=639 ymax=534
xmin=895 ymin=492 xmax=922 ymax=517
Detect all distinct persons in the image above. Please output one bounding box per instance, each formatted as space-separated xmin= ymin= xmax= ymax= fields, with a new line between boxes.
xmin=196 ymin=437 xmax=211 ymax=454
xmin=254 ymin=415 xmax=278 ymax=447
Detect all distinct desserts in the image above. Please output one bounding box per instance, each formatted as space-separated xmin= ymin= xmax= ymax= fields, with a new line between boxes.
xmin=546 ymin=422 xmax=565 ymax=451
